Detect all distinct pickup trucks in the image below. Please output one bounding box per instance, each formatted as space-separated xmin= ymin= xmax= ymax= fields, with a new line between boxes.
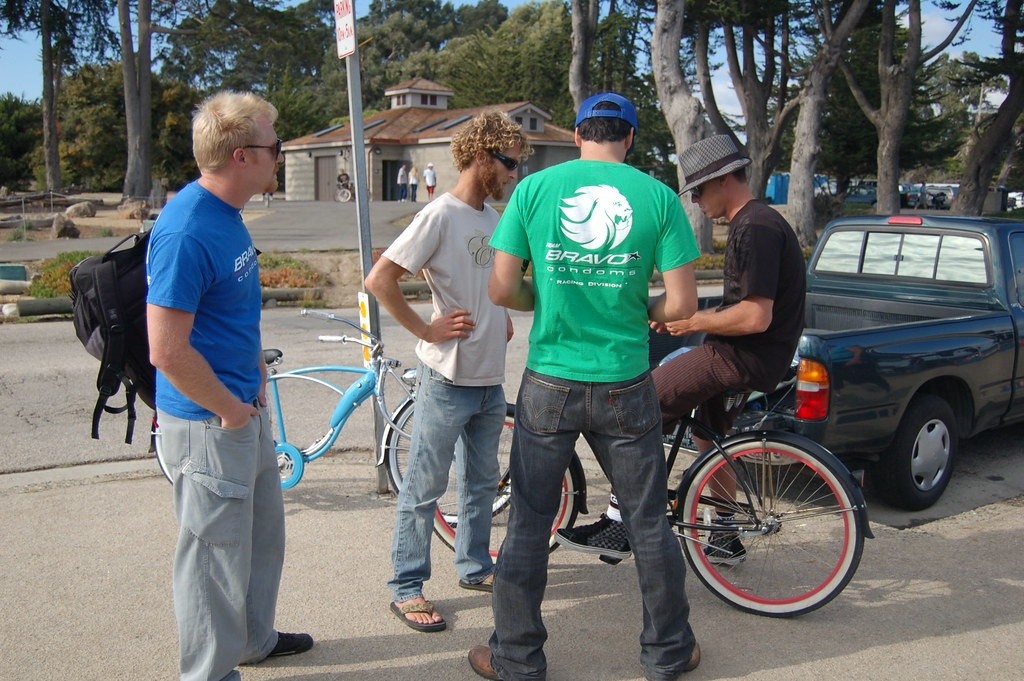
xmin=655 ymin=216 xmax=1024 ymax=513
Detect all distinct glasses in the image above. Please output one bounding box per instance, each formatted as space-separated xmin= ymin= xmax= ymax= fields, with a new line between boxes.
xmin=488 ymin=149 xmax=519 ymax=170
xmin=233 ymin=140 xmax=281 ymax=160
xmin=690 ymin=186 xmax=703 ymax=197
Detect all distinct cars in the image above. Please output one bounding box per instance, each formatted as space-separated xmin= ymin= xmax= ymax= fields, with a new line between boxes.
xmin=814 ymin=176 xmax=960 ymax=209
xmin=1007 ymin=192 xmax=1024 ymax=211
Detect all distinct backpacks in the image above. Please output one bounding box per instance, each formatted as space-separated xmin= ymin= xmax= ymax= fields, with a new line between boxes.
xmin=68 ymin=228 xmax=157 ymax=410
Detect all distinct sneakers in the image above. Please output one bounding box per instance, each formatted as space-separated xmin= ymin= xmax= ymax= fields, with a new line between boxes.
xmin=554 ymin=513 xmax=632 ymax=559
xmin=267 ymin=632 xmax=313 ymax=656
xmin=703 ymin=532 xmax=747 ymax=565
xmin=683 ymin=642 xmax=700 ymax=671
xmin=468 ymin=645 xmax=501 ymax=681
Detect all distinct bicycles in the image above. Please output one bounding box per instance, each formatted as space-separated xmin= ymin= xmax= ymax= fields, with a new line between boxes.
xmin=149 ymin=308 xmax=520 ymax=528
xmin=431 ymin=383 xmax=876 ymax=619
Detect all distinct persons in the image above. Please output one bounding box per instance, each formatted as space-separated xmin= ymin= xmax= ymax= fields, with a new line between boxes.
xmin=554 ymin=135 xmax=807 ymax=566
xmin=337 ymin=169 xmax=349 ymax=189
xmin=265 ymin=193 xmax=273 ymax=207
xmin=422 ymin=163 xmax=436 ymax=202
xmin=364 ymin=113 xmax=534 ymax=631
xmin=914 ymin=181 xmax=930 ymax=211
xmin=468 ymin=93 xmax=703 ymax=680
xmin=408 ymin=167 xmax=419 ymax=202
xmin=146 ymin=93 xmax=314 ymax=681
xmin=397 ymin=163 xmax=407 ymax=203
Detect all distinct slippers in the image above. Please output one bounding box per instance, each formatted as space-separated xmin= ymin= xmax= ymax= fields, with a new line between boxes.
xmin=460 ymin=576 xmax=493 ymax=592
xmin=390 ymin=600 xmax=446 ymax=631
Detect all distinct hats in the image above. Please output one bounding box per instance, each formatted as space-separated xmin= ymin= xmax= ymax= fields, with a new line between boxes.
xmin=427 ymin=163 xmax=434 ymax=167
xmin=576 ymin=93 xmax=638 ymax=155
xmin=677 ymin=134 xmax=752 ymax=198
